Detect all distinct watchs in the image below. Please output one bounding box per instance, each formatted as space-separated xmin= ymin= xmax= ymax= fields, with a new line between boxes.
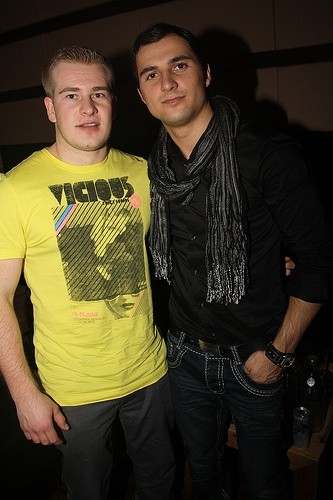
xmin=265 ymin=342 xmax=295 ymax=369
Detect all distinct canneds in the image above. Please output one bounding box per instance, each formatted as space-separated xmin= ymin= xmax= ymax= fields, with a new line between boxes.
xmin=292 ymin=406 xmax=311 ymax=448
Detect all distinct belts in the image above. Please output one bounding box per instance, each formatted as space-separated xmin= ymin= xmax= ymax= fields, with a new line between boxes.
xmin=167 ymin=322 xmax=274 ymax=358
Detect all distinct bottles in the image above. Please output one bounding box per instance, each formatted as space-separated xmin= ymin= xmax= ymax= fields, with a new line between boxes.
xmin=300 ymin=356 xmax=327 ymax=415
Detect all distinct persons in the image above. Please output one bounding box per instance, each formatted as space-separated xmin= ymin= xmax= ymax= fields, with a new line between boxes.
xmin=2 ymin=46 xmax=179 ymax=500
xmin=134 ymin=22 xmax=330 ymax=500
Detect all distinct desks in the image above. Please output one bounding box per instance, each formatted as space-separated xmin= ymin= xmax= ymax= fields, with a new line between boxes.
xmin=225 ymin=360 xmax=333 ymax=500
